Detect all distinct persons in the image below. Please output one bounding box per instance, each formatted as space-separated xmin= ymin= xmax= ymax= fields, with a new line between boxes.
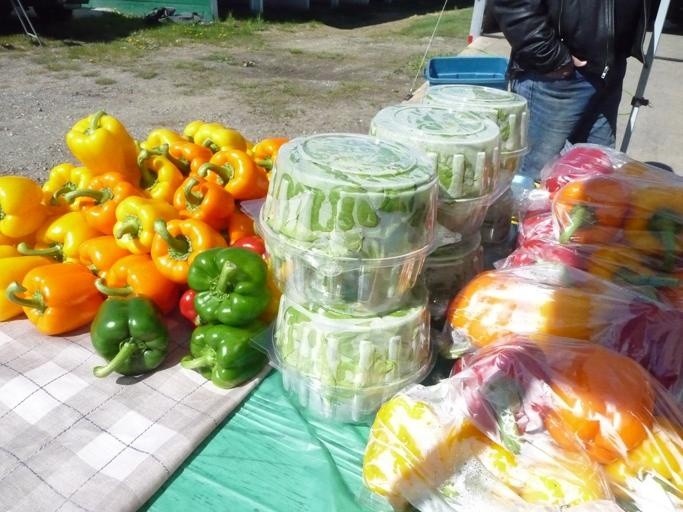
xmin=488 ymin=0 xmax=646 ymax=182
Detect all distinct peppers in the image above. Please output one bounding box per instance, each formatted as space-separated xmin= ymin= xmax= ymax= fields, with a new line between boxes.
xmin=359 ymin=140 xmax=681 ymax=512
xmin=0 ymin=107 xmax=293 ymax=392
